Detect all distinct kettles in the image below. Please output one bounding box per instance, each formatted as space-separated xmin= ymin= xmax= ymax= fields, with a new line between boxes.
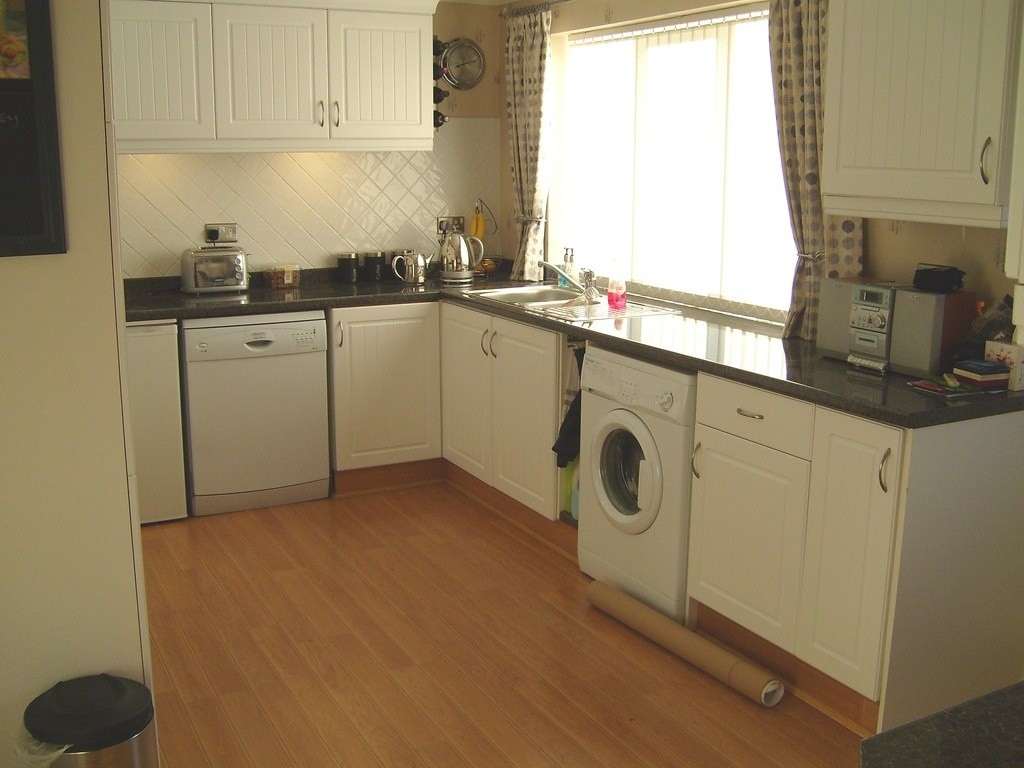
xmin=438 ymin=224 xmax=484 ymax=286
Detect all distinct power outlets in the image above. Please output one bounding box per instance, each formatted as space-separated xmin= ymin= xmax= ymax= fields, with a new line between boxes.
xmin=436 ymin=217 xmax=465 ymax=235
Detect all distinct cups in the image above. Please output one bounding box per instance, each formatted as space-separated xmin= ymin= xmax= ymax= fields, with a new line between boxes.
xmin=366 ymin=252 xmax=385 ymax=282
xmin=392 ymin=249 xmax=411 ymax=281
xmin=338 ymin=253 xmax=359 ymax=283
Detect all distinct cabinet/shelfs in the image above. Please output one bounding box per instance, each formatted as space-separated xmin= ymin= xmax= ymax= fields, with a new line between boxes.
xmin=793 ymin=403 xmax=906 ymax=706
xmin=329 ymin=302 xmax=441 ymax=472
xmin=820 ymin=0 xmax=1024 ymax=230
xmin=124 ymin=324 xmax=187 ymax=524
xmin=107 ymin=0 xmax=215 ymax=154
xmin=212 ymin=3 xmax=433 ymax=152
xmin=441 ymin=300 xmax=562 ymax=522
xmin=687 ymin=372 xmax=816 ymax=656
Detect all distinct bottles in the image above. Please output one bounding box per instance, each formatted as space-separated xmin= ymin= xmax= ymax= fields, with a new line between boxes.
xmin=608 ymin=258 xmax=626 ymax=308
xmin=433 ymin=39 xmax=450 ymax=56
xmin=433 ymin=63 xmax=449 ymax=80
xmin=434 ymin=110 xmax=450 ymax=128
xmin=433 ymin=85 xmax=450 ymax=104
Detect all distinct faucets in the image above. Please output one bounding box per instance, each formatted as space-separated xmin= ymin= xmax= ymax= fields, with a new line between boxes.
xmin=538 ymin=261 xmax=596 ymax=294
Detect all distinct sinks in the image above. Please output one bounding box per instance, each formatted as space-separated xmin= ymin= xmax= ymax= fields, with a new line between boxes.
xmin=459 ymin=284 xmax=577 ymax=305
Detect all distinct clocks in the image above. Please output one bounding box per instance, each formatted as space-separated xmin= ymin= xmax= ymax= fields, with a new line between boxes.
xmin=441 ymin=38 xmax=485 ymax=90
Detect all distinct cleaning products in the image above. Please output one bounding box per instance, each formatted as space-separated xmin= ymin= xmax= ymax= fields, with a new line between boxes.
xmin=608 ymin=276 xmax=627 ymax=310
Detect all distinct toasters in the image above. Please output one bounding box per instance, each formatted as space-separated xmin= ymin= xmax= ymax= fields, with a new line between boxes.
xmin=181 ymin=244 xmax=249 ymax=298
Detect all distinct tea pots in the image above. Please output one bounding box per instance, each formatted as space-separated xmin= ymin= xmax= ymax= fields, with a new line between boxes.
xmin=393 ymin=250 xmax=435 ymax=285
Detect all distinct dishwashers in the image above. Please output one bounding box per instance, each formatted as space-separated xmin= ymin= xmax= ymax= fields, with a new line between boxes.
xmin=182 ymin=310 xmax=330 ymax=517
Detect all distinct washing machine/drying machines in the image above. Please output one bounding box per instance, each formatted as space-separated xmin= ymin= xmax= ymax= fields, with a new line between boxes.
xmin=577 ymin=339 xmax=698 ymax=623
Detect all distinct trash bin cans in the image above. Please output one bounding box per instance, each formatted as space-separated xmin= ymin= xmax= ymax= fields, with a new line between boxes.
xmin=20 ymin=673 xmax=159 ymax=768
